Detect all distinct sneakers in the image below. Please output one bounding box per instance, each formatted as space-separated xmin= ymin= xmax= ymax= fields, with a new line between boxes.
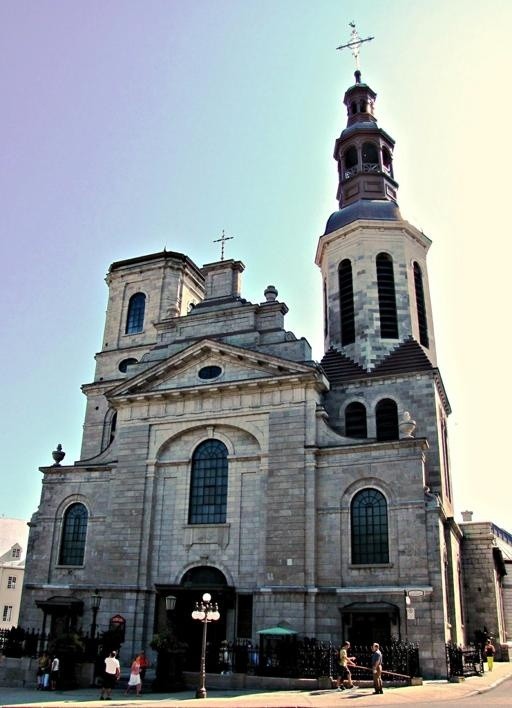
xmin=124 ymin=691 xmax=144 ymax=697
xmin=100 ymin=697 xmax=104 ymax=700
xmin=348 ymin=685 xmax=353 ymax=689
xmin=489 ymin=668 xmax=492 ymax=672
xmin=340 ymin=686 xmax=344 ymax=690
xmin=36 ymin=688 xmax=56 ymax=691
xmin=105 ymin=697 xmax=112 ymax=701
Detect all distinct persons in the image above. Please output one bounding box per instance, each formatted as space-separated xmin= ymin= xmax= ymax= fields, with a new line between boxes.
xmin=35 ymin=650 xmax=48 ymax=688
xmin=137 ymin=648 xmax=149 ymax=694
xmin=100 ymin=649 xmax=120 ymax=699
xmin=335 ymin=643 xmax=357 ymax=690
xmin=335 ymin=640 xmax=360 ymax=691
xmin=124 ymin=653 xmax=142 ymax=697
xmin=225 ymin=639 xmax=235 ymax=673
xmin=233 ymin=638 xmax=288 ymax=674
xmin=218 ymin=638 xmax=229 ymax=675
xmin=371 ymin=641 xmax=385 ymax=694
xmin=49 ymin=653 xmax=59 ymax=689
xmin=40 ymin=654 xmax=52 ymax=689
xmin=483 ymin=638 xmax=496 ymax=672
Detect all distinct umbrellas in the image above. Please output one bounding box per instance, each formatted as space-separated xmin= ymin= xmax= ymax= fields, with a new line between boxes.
xmin=256 ymin=624 xmax=300 ymax=636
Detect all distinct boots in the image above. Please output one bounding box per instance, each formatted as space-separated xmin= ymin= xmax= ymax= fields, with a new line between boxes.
xmin=372 ymin=687 xmax=379 ymax=694
xmin=380 ymin=686 xmax=383 ymax=694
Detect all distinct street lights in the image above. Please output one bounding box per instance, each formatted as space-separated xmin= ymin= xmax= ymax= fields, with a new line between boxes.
xmin=192 ymin=592 xmax=222 ymax=698
xmin=89 ymin=587 xmax=103 ymax=637
xmin=403 ymin=589 xmax=411 ymax=643
